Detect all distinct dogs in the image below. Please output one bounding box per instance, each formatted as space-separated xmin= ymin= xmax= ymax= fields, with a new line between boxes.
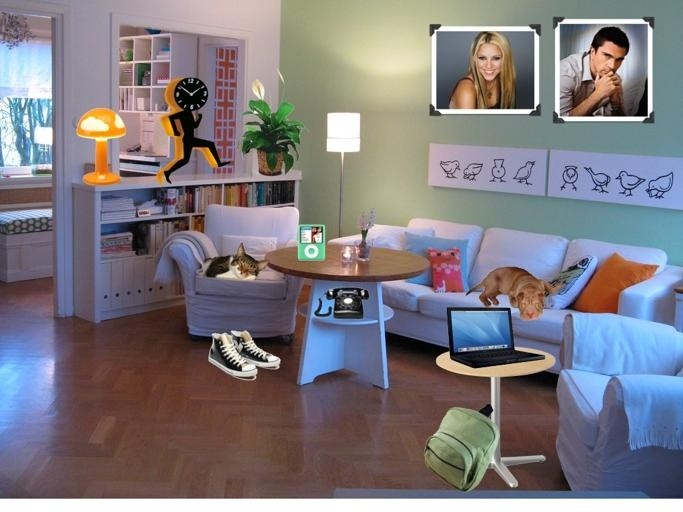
xmin=465 ymin=266 xmax=563 ymax=322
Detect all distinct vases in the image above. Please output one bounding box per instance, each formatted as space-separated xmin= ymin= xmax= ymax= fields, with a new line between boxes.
xmin=254 ymin=146 xmax=282 ymax=176
xmin=356 ymin=239 xmax=371 ymax=261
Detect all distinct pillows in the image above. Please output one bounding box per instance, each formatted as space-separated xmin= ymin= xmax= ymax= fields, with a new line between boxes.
xmin=575 ymin=250 xmax=660 ymax=314
xmin=538 ymin=255 xmax=597 ymax=312
xmin=404 ymin=231 xmax=469 ymax=295
xmin=222 ymin=234 xmax=279 ymax=267
xmin=361 ymin=224 xmax=435 ymax=255
xmin=424 ymin=246 xmax=466 ymax=294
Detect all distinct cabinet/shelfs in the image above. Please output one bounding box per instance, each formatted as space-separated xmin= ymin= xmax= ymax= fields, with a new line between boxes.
xmin=117 ymin=33 xmax=198 ymax=175
xmin=71 ymin=171 xmax=304 ymax=325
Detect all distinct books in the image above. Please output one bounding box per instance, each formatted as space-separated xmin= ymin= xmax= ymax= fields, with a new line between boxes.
xmin=119 ymin=37 xmax=170 ymax=157
xmin=99 ymin=179 xmax=295 ymax=262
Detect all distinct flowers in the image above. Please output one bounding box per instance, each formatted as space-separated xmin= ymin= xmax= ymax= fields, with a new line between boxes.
xmin=241 ymin=68 xmax=305 ymax=174
xmin=354 ymin=207 xmax=378 ymax=260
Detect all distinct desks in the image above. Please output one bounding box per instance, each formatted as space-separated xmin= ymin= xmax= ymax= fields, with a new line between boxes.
xmin=264 ymin=243 xmax=431 ymax=391
xmin=431 ymin=345 xmax=557 ymax=490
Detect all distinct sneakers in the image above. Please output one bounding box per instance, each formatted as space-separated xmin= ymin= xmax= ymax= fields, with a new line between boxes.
xmin=231 ymin=330 xmax=280 ymax=367
xmin=208 ymin=333 xmax=258 ymax=377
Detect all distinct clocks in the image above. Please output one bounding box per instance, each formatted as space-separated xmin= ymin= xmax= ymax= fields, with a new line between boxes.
xmin=174 ymin=77 xmax=208 ymax=111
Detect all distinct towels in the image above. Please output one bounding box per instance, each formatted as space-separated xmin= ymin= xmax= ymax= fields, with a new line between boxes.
xmin=153 ymin=229 xmax=218 ymax=288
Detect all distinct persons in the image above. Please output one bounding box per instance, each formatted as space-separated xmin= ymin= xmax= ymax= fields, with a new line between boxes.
xmin=558 ymin=25 xmax=634 ymax=117
xmin=443 ymin=30 xmax=520 ymax=110
xmin=310 ymin=227 xmax=323 ymax=243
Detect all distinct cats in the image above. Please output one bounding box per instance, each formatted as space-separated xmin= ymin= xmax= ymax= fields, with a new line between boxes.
xmin=201 ymin=243 xmax=268 ymax=282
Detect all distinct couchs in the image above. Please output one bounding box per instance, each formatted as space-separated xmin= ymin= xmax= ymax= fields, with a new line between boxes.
xmin=166 ymin=203 xmax=306 ymax=345
xmin=327 ymin=218 xmax=682 ymax=379
xmin=552 ymin=313 xmax=681 ymax=497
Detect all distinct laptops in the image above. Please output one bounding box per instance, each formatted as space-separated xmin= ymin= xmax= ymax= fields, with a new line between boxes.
xmin=447 ymin=307 xmax=545 ymax=368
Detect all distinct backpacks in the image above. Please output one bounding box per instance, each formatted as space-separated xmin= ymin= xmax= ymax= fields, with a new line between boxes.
xmin=423 ymin=404 xmax=501 ymax=493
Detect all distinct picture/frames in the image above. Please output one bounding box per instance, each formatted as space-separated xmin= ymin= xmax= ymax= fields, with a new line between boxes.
xmin=550 ymin=14 xmax=655 ymax=124
xmin=428 ymin=22 xmax=543 ymax=117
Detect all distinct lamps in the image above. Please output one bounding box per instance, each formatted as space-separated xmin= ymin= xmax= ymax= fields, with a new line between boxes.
xmin=33 ymin=125 xmax=52 ymax=174
xmin=75 ymin=106 xmax=128 ymax=186
xmin=325 ymin=112 xmax=360 ymax=237
